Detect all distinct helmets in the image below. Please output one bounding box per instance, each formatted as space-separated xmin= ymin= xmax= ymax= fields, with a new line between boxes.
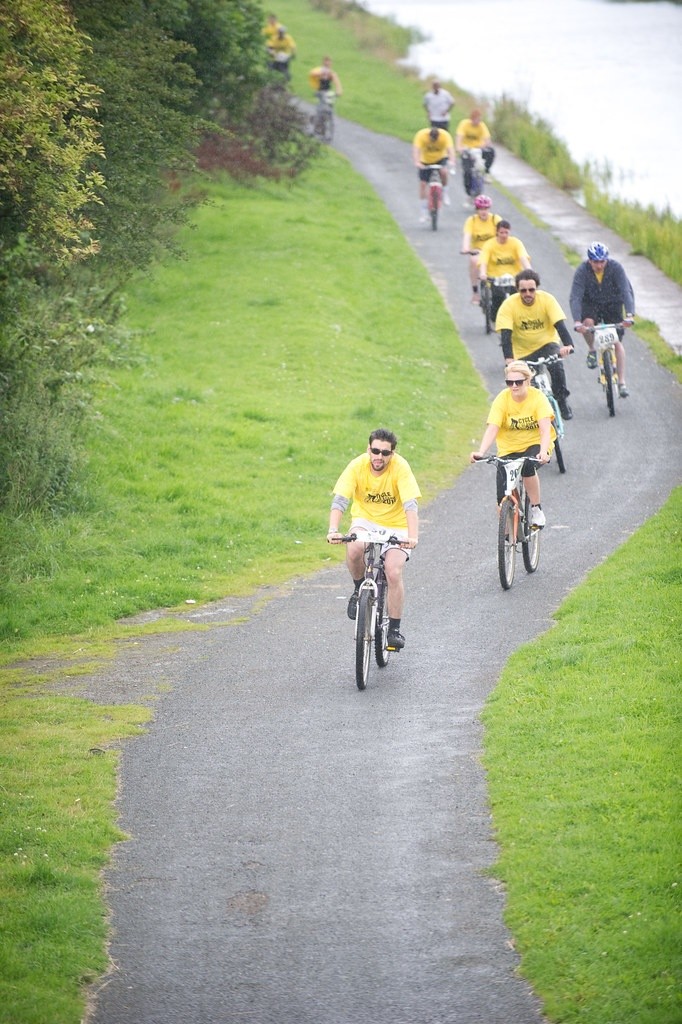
xmin=588 ymin=242 xmax=609 ymax=263
xmin=475 ymin=194 xmax=492 ymax=209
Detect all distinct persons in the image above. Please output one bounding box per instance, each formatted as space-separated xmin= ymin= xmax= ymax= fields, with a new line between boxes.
xmin=456 ymin=110 xmax=495 ymax=196
xmin=327 ymin=428 xmax=422 ymax=648
xmin=495 ymin=268 xmax=574 ymax=421
xmin=414 ymin=127 xmax=455 ymax=214
xmin=310 ymin=55 xmax=342 ymax=112
xmin=469 ymin=360 xmax=556 ymax=542
xmin=462 ymin=194 xmax=502 ymax=305
xmin=422 ymin=79 xmax=455 ymax=130
xmin=568 ymin=242 xmax=635 ymax=397
xmin=477 ymin=220 xmax=532 ymax=320
xmin=260 ymin=13 xmax=296 ymax=75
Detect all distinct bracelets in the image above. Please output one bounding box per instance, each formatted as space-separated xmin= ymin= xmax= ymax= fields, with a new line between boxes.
xmin=626 ymin=313 xmax=632 ymax=317
xmin=329 ymin=528 xmax=338 ymax=533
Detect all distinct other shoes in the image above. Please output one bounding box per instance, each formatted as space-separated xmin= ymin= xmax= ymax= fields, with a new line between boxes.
xmin=472 ymin=292 xmax=481 ymax=305
xmin=441 ymin=191 xmax=451 ymax=205
xmin=490 ymin=305 xmax=498 ymax=322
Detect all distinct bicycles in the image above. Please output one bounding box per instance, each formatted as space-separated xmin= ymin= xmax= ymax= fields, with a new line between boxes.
xmin=422 ymin=165 xmax=452 ymax=232
xmin=474 ymin=454 xmax=546 ymax=591
xmin=327 ymin=533 xmax=416 ymax=689
xmin=576 ymin=320 xmax=635 ymax=417
xmin=458 ymin=146 xmax=492 ymax=198
xmin=311 ymin=92 xmax=338 ymax=142
xmin=461 ymin=251 xmax=519 ymax=338
xmin=506 ymin=348 xmax=574 ymax=474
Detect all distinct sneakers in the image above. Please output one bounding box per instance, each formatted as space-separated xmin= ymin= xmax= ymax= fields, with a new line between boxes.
xmin=586 ymin=350 xmax=598 ymax=368
xmin=619 ymin=383 xmax=629 ymax=397
xmin=347 ymin=587 xmax=359 ymax=620
xmin=530 ymin=506 xmax=545 ymax=526
xmin=559 ymin=398 xmax=573 ymax=419
xmin=386 ymin=626 xmax=405 ymax=648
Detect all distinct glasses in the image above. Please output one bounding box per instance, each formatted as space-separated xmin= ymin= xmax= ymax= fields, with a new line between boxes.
xmin=505 ymin=377 xmax=528 ymax=387
xmin=591 ymin=259 xmax=607 ymax=264
xmin=370 ymin=444 xmax=393 ymax=457
xmin=518 ymin=288 xmax=537 ymax=294
